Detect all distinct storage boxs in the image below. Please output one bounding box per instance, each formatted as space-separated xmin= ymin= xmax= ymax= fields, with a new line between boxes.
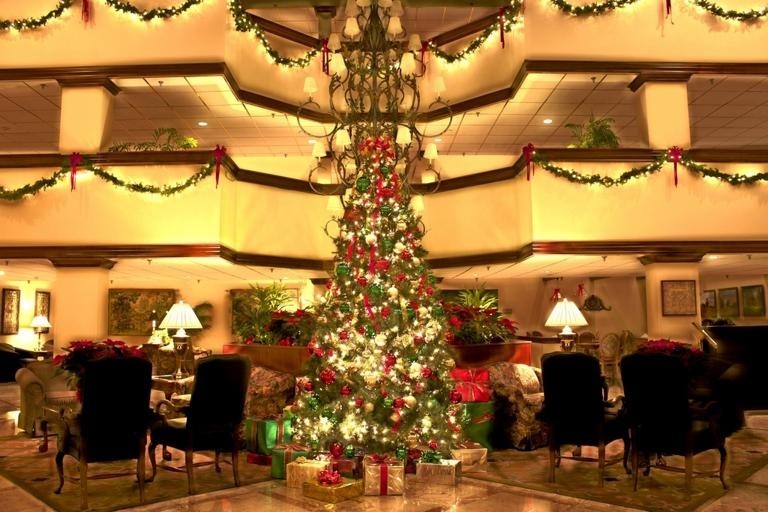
xmin=245 ymin=366 xmax=490 ymax=505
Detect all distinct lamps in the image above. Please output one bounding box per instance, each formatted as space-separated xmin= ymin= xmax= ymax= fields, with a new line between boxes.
xmin=544 ymin=296 xmax=590 ymax=352
xmin=159 ymin=298 xmax=205 ymax=378
xmin=30 ymin=316 xmax=50 ymax=349
xmin=298 ymin=0 xmax=442 ymax=218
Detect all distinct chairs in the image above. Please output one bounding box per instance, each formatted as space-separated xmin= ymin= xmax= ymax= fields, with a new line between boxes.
xmin=147 ymin=351 xmax=254 ymax=496
xmin=616 ymin=351 xmax=733 ymax=494
xmin=52 ymin=358 xmax=153 ymax=510
xmin=540 ymin=350 xmax=634 ymax=488
xmin=571 ymin=329 xmax=632 ymax=380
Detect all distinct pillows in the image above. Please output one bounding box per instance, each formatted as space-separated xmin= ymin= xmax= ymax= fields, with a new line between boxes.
xmin=510 ymin=363 xmax=540 ymax=394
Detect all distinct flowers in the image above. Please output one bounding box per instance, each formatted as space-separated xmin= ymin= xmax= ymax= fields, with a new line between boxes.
xmin=228 ymin=278 xmax=318 ymax=347
xmin=54 ymin=339 xmax=146 ymax=402
xmin=439 ymin=277 xmax=520 ymax=345
xmin=638 ymin=336 xmax=705 ymax=362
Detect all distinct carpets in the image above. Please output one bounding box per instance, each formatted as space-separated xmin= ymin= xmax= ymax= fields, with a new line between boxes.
xmin=457 ymin=430 xmax=766 ymax=511
xmin=1 ymin=446 xmax=274 ymax=511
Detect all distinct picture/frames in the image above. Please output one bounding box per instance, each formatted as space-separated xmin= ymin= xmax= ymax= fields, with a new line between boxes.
xmin=1 ymin=285 xmax=21 ymax=336
xmin=108 ymin=286 xmax=180 ymax=335
xmin=741 ymin=284 xmax=767 ymax=319
xmin=700 ymin=288 xmax=718 ymax=318
xmin=717 ymin=286 xmax=741 ymax=318
xmin=32 ymin=289 xmax=54 ymax=333
xmin=659 ymin=278 xmax=698 ymax=317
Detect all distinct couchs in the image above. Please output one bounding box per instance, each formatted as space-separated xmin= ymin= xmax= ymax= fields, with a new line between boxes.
xmin=170 ymin=367 xmax=298 ymax=450
xmin=0 ymin=342 xmax=33 ymax=381
xmin=475 ymin=359 xmax=546 ymax=451
xmin=14 ymin=361 xmax=83 ymax=437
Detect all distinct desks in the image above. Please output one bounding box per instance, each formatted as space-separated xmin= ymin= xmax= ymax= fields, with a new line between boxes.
xmin=33 ymin=350 xmax=54 ymax=360
xmin=151 ymin=373 xmax=195 ymax=395
